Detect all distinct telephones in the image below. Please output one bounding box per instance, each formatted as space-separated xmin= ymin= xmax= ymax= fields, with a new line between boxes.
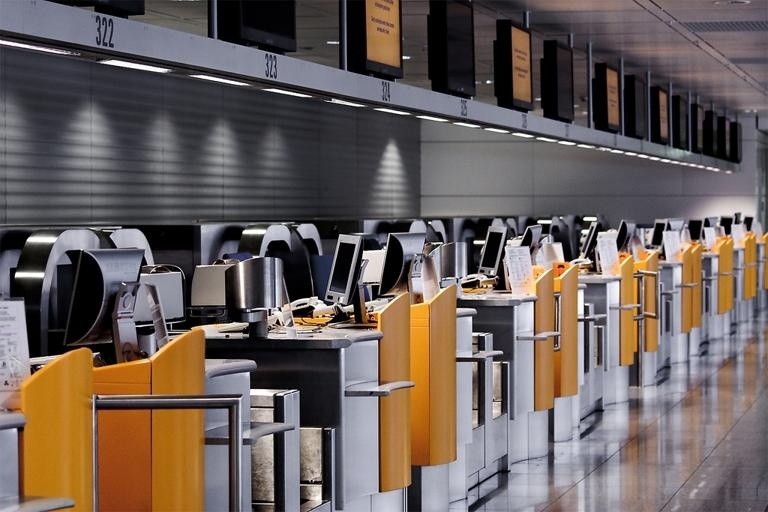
xmin=460 ymin=274 xmax=488 ymax=288
xmin=570 ymin=258 xmax=591 ymax=269
xmin=283 ymin=296 xmax=328 ymax=318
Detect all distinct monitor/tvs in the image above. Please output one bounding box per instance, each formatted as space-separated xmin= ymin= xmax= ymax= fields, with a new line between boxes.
xmin=377 ymin=232 xmax=428 ymax=296
xmin=617 ymin=219 xmax=637 ymax=253
xmin=651 ymin=213 xmax=753 ymax=248
xmin=521 ymin=225 xmax=543 ymax=255
xmin=477 ymin=226 xmax=508 ymax=290
xmin=324 ymin=234 xmax=378 ymax=329
xmin=581 ymin=221 xmax=602 ymax=272
xmin=63 ymin=248 xmax=145 ymax=346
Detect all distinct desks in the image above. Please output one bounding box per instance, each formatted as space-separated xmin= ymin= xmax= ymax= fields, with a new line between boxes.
xmin=658 ymin=262 xmax=683 ymax=380
xmin=733 ymin=246 xmax=744 ymax=333
xmin=455 ymin=290 xmax=536 ymax=502
xmin=578 ymin=275 xmax=621 ymax=427
xmin=206 ymin=318 xmax=379 ymax=510
xmin=703 ymin=254 xmax=721 ymax=345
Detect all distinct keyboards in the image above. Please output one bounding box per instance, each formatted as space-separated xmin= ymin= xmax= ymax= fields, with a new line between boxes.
xmin=218 ymin=315 xmax=278 ymax=333
xmin=343 ymin=297 xmax=388 ymax=311
xmin=29 ymin=351 xmax=99 ymax=367
